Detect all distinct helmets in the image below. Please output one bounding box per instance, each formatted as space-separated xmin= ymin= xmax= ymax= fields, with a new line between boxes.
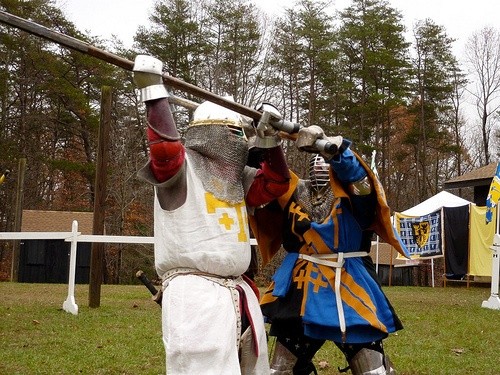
xmin=310 ymin=153 xmax=330 ymax=192
xmin=188 ymin=99 xmax=244 ymax=129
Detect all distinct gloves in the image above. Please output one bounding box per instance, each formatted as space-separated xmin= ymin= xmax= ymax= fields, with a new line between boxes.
xmin=293 ymin=125 xmax=343 ymax=161
xmin=131 ymin=56 xmax=170 ymax=103
xmin=248 ymin=105 xmax=283 ymax=149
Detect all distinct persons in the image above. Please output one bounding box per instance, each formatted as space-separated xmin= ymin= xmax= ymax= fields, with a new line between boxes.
xmin=133 ymin=55 xmax=291 ymax=375
xmin=242 ymin=124 xmax=412 ymax=375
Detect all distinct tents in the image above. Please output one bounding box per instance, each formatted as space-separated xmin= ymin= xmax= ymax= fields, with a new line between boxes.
xmin=376 ymin=190 xmax=476 ymax=288
